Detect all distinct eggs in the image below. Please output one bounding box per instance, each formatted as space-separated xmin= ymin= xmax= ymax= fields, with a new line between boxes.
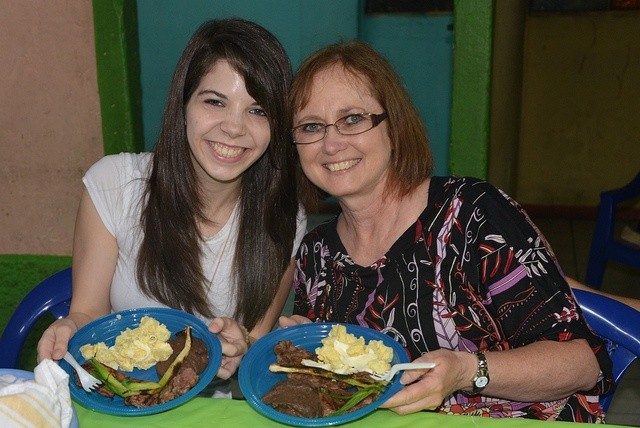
xmin=80 ymin=315 xmax=174 ymax=372
xmin=302 ymin=323 xmax=392 ymax=377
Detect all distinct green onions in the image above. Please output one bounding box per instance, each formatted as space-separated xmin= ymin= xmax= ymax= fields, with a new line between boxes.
xmin=269 ymin=364 xmax=393 ymax=418
xmin=87 ymin=325 xmax=193 ymax=398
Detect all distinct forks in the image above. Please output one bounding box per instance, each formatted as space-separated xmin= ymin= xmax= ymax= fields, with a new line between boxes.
xmin=62 ymin=351 xmax=102 ymax=393
xmin=369 ymin=362 xmax=436 ymax=382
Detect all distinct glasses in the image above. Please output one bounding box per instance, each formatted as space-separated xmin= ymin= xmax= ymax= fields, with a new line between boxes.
xmin=289 ymin=111 xmax=387 ymax=144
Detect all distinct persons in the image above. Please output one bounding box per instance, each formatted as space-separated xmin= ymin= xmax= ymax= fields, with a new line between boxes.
xmin=35 ymin=17 xmax=309 ymax=400
xmin=276 ymin=40 xmax=617 ymax=424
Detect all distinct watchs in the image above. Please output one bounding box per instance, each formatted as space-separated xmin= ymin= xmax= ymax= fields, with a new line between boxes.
xmin=462 ymin=352 xmax=490 ymax=396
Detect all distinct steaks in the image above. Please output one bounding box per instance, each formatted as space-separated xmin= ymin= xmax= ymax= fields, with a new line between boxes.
xmin=261 ymin=341 xmax=373 ymax=419
xmin=74 ymin=332 xmax=209 ymax=408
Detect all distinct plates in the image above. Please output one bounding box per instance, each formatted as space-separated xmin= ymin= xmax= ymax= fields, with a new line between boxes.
xmin=58 ymin=307 xmax=222 ymax=418
xmin=237 ymin=321 xmax=409 ymax=428
xmin=0 ymin=368 xmax=81 ymax=428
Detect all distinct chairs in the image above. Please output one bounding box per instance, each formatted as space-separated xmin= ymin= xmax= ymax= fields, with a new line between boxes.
xmin=580 ymin=173 xmax=640 ymax=289
xmin=3 ymin=268 xmax=71 ymax=369
xmin=572 ymin=285 xmax=640 ymax=396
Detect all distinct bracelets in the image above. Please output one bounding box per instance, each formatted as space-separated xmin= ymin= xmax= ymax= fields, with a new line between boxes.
xmin=240 ymin=326 xmax=249 ymax=344
